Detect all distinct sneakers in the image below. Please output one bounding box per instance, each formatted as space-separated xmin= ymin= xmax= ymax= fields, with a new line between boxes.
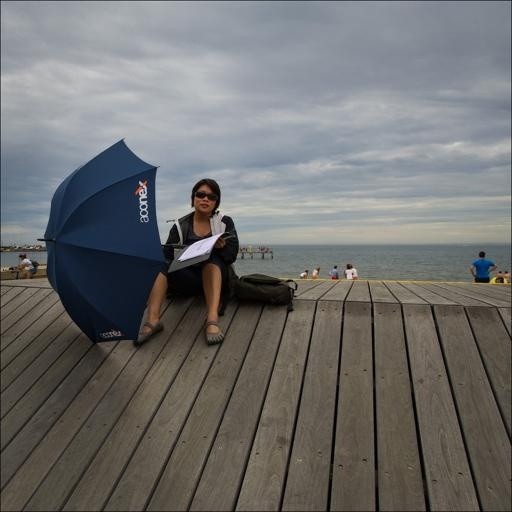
xmin=204 ymin=321 xmax=224 ymax=345
xmin=133 ymin=322 xmax=163 ymax=347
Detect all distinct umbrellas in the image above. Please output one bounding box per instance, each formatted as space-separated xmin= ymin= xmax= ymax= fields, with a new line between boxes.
xmin=32 ymin=136 xmax=188 ymax=346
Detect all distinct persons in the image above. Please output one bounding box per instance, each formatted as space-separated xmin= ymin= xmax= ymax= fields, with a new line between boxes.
xmin=299 ymin=269 xmax=309 ymax=279
xmin=497 ymin=270 xmax=511 ymax=283
xmin=344 ymin=266 xmax=353 ymax=280
xmin=132 ymin=179 xmax=240 ymax=344
xmin=328 ymin=265 xmax=340 ymax=280
xmin=348 ymin=264 xmax=358 ymax=280
xmin=311 ymin=267 xmax=321 ymax=279
xmin=240 ymin=244 xmax=272 ymax=252
xmin=469 ymin=250 xmax=498 ymax=283
xmin=14 ymin=254 xmax=36 ymax=280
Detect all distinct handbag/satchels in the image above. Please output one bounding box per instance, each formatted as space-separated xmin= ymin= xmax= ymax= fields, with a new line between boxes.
xmin=237 ymin=273 xmax=294 ymax=306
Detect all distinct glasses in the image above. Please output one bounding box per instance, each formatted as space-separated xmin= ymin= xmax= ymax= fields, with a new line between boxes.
xmin=195 ymin=191 xmax=218 ymax=200
